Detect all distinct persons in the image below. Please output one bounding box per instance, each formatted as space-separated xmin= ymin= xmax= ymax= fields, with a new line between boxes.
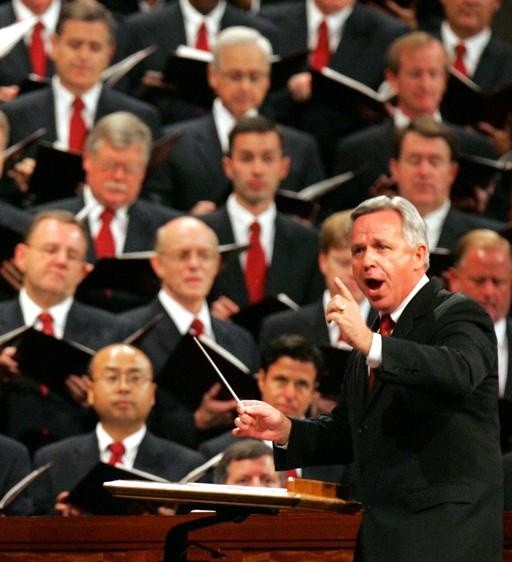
xmin=1 ymin=1 xmax=511 ymax=517
xmin=232 ymin=196 xmax=502 ymax=562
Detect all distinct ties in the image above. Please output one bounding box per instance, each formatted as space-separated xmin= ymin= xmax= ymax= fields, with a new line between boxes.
xmin=191 ymin=320 xmax=203 ymax=338
xmin=454 ymin=45 xmax=467 ymax=76
xmin=93 ymin=211 xmax=114 ymax=258
xmin=69 ymin=99 xmax=88 ymax=154
xmin=195 ymin=27 xmax=209 ymax=51
xmin=368 ymin=314 xmax=391 ymax=388
xmin=243 ymin=225 xmax=267 ymax=301
xmin=30 ymin=21 xmax=46 ymax=76
xmin=107 ymin=442 xmax=124 ymax=467
xmin=312 ymin=23 xmax=328 ymax=67
xmin=35 ymin=314 xmax=54 ymax=395
